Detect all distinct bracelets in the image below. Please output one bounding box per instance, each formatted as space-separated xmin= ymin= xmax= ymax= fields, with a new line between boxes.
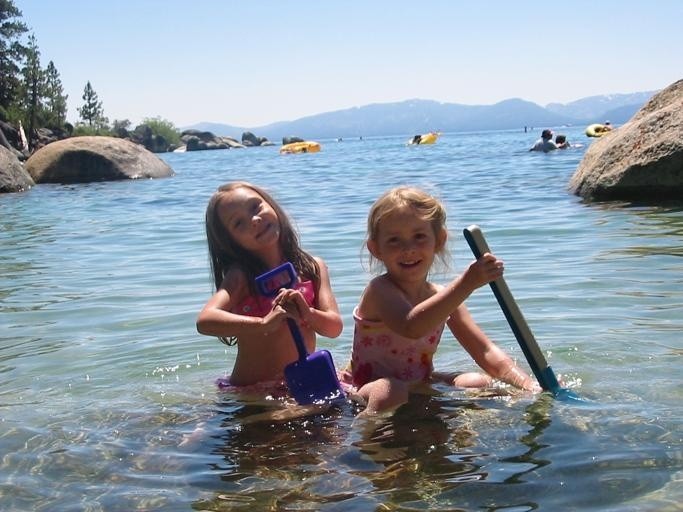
xmin=500 ymin=364 xmax=528 ymax=388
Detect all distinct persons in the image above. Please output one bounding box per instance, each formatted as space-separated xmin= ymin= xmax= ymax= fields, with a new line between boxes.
xmin=553 ymin=134 xmax=584 ymax=151
xmin=338 ymin=185 xmax=566 ymax=440
xmin=596 ymin=120 xmax=613 ymax=133
xmin=409 ymin=135 xmax=422 ymax=145
xmin=280 ymin=148 xmax=294 ymax=155
xmin=528 ymin=127 xmax=561 ymax=153
xmin=194 ymin=180 xmax=343 ymax=402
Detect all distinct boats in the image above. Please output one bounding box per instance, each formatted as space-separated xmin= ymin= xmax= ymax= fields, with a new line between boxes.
xmin=409 ymin=132 xmax=439 ymax=146
xmin=281 ymin=140 xmax=320 ymax=156
xmin=585 ymin=123 xmax=612 ymax=138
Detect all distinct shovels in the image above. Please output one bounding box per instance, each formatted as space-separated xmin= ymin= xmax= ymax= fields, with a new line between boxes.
xmin=256 ymin=262 xmax=345 ymax=405
